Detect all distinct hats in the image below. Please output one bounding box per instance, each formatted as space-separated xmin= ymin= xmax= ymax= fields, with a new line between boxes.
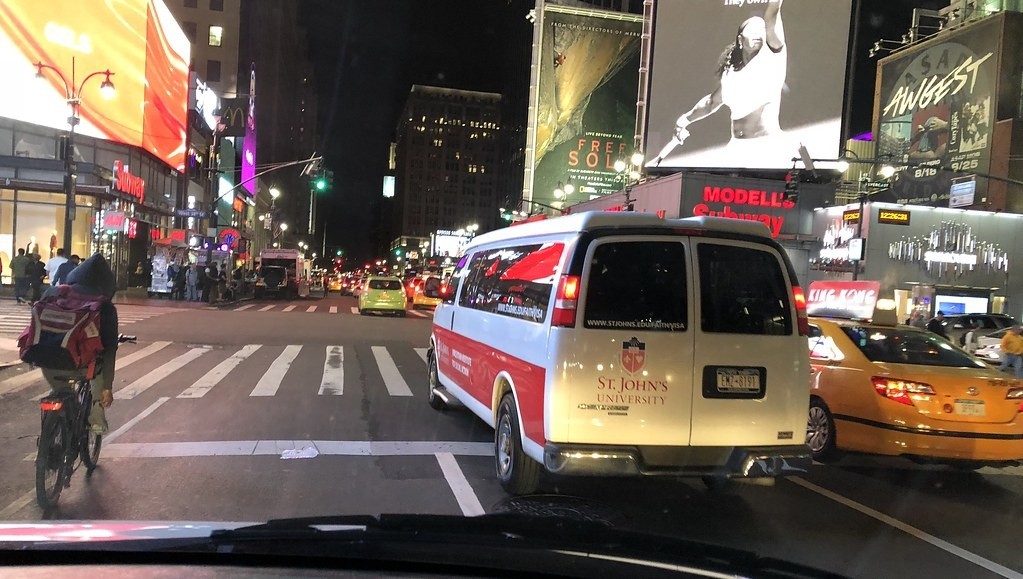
xmin=937 ymin=311 xmax=945 ymax=315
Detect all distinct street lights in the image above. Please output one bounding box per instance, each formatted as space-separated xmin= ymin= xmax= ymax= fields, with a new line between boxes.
xmin=30 ymin=57 xmax=116 ymax=256
xmin=834 ymin=145 xmax=897 ymax=282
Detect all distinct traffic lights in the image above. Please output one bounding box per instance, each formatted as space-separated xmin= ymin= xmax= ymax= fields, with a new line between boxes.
xmin=314 ymin=177 xmax=327 ymax=192
xmin=336 ymin=250 xmax=343 ymax=257
xmin=338 ymin=259 xmax=343 ymax=264
xmin=324 ymin=170 xmax=335 ymax=190
xmin=395 ymin=249 xmax=402 ymax=256
xmin=309 ymin=170 xmax=318 ymax=189
xmin=503 ymin=199 xmax=511 ymax=221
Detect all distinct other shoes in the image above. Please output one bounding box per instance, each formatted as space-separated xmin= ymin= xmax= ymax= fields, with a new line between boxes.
xmin=87 ymin=402 xmax=108 ymax=435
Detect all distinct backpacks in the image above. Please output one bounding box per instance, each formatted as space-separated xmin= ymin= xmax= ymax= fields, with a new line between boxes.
xmin=960 ymin=331 xmax=975 ymax=346
xmin=16 ymin=284 xmax=110 ymax=379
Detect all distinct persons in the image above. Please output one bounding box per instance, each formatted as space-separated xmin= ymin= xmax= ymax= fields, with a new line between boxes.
xmin=323 ymin=278 xmax=330 ymax=297
xmin=671 ymin=0 xmax=792 ymax=162
xmin=909 ymin=99 xmax=989 ymax=161
xmin=928 ymin=311 xmax=945 ymax=338
xmin=999 ymin=324 xmax=1023 ymax=380
xmin=965 ymin=322 xmax=978 ymax=354
xmin=43 ymin=248 xmax=85 ymax=287
xmin=41 ymin=254 xmax=118 ymax=437
xmin=134 ymin=259 xmax=258 ymax=303
xmin=9 ymin=243 xmax=46 ymax=305
xmin=910 ymin=311 xmax=924 ymax=327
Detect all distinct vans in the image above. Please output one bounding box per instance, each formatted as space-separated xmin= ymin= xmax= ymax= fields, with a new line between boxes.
xmin=425 ymin=211 xmax=810 ymax=495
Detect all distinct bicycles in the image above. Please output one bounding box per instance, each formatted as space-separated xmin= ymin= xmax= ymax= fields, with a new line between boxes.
xmin=36 ymin=333 xmax=136 ymax=509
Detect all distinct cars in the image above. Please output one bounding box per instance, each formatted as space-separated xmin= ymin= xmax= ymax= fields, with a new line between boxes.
xmin=921 ymin=312 xmax=1023 ymax=365
xmin=324 ymin=266 xmax=446 ymax=318
xmin=808 ymin=316 xmax=1023 ymax=470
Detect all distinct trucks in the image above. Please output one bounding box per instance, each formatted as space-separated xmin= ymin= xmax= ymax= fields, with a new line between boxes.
xmin=252 ymin=249 xmax=306 ymax=299
